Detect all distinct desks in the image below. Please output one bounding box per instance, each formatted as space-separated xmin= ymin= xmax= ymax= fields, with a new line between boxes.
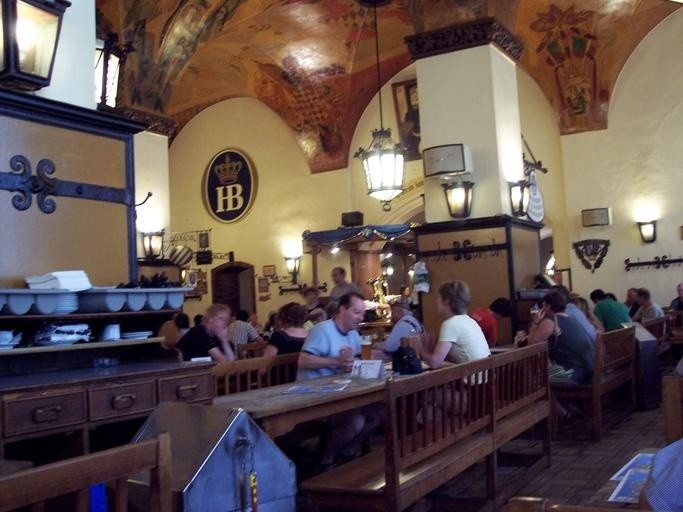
xmin=662 ymin=357 xmax=682 ymax=444
xmin=214 ymin=359 xmax=456 ymax=439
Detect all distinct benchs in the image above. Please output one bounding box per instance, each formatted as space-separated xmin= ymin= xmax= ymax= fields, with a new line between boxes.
xmin=644 ymin=317 xmax=670 ymax=356
xmin=550 ymin=326 xmax=637 ymax=424
xmin=3 ymin=432 xmax=171 ymax=512
xmin=297 ymin=339 xmax=551 ymax=512
xmin=220 ymin=340 xmax=304 ymax=396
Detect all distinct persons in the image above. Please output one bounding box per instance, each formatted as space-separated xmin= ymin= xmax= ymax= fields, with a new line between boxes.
xmin=258 ymin=302 xmax=308 ymax=388
xmin=639 ymin=438 xmax=682 ymax=512
xmin=294 ymin=292 xmax=366 ymax=470
xmin=157 ymin=268 xmax=427 ymax=363
xmin=410 ymin=280 xmax=496 ymax=430
xmin=528 ymin=285 xmax=683 ymax=430
xmin=467 ymin=297 xmax=513 ymax=348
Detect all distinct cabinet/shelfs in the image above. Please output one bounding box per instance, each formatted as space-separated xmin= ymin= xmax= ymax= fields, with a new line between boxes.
xmin=0 ymin=307 xmax=217 ymax=456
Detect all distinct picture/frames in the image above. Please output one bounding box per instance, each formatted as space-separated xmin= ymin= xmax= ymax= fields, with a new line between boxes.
xmin=262 ymin=264 xmax=277 ymax=277
xmin=257 ymin=278 xmax=269 ymax=293
xmin=201 ymin=148 xmax=258 ymax=224
xmin=392 ymin=79 xmax=421 ymax=160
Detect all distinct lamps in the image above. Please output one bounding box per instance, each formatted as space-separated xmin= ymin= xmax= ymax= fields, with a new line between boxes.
xmin=438 ymin=182 xmax=477 ymax=219
xmin=421 ymin=142 xmax=475 ymax=182
xmin=284 ymin=257 xmax=302 ymax=275
xmin=139 ymin=228 xmax=169 ymax=260
xmin=507 ymin=179 xmax=534 ymax=217
xmin=95 ymin=38 xmax=128 ymax=114
xmin=580 ymin=206 xmax=613 ymax=228
xmin=1 ymin=0 xmax=73 ymax=94
xmin=352 ymin=0 xmax=415 ymax=213
xmin=636 ymin=220 xmax=659 ymax=244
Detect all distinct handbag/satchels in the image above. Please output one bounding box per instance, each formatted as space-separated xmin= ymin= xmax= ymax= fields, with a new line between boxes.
xmin=392 ymin=346 xmax=421 ymax=374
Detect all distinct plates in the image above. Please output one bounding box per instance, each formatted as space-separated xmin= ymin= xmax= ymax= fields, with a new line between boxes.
xmin=0 ymin=342 xmax=19 ymax=350
xmin=121 ymin=332 xmax=152 ymax=340
xmin=54 ymin=294 xmax=80 ymax=314
